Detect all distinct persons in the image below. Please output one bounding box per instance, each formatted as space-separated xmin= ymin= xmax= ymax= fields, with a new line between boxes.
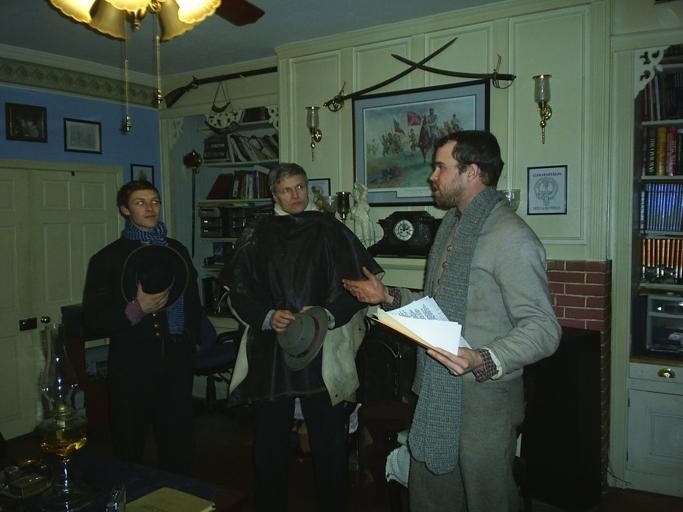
xmin=451 ymin=113 xmax=459 ymax=128
xmin=25 ymin=117 xmax=40 ymax=137
xmin=341 ymin=129 xmax=562 ymax=512
xmin=223 ymin=163 xmax=386 ymax=474
xmin=81 ymin=180 xmax=203 ymax=462
xmin=367 ymin=128 xmax=419 ymax=156
xmin=426 ymin=108 xmax=437 ymax=133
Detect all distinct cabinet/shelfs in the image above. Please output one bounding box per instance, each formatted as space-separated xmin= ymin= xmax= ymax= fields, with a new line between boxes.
xmin=157 ymin=92 xmax=281 ymax=319
xmin=606 ymin=27 xmax=683 ymax=501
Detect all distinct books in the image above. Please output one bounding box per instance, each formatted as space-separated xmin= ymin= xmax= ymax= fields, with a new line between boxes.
xmin=644 ymin=127 xmax=683 ymax=177
xmin=206 ymin=170 xmax=271 ymax=200
xmin=641 ymin=240 xmax=683 ymax=287
xmin=202 ymin=240 xmax=232 ymax=269
xmin=640 ymin=183 xmax=683 ymax=233
xmin=370 ymin=294 xmax=472 ymax=362
xmin=647 ymin=73 xmax=683 ymax=118
xmin=203 ymin=132 xmax=279 ymax=163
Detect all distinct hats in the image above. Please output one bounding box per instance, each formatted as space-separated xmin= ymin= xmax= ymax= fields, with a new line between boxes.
xmin=277 ymin=307 xmax=328 ymax=370
xmin=120 ymin=244 xmax=190 ymax=311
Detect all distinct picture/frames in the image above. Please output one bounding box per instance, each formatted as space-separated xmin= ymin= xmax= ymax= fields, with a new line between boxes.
xmin=4 ymin=102 xmax=49 ymax=143
xmin=351 ymin=78 xmax=491 ymax=207
xmin=131 ymin=164 xmax=154 ymax=184
xmin=63 ymin=117 xmax=102 ymax=155
xmin=309 ymin=178 xmax=331 ymax=196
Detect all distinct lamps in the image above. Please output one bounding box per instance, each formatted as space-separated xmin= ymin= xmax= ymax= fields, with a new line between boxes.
xmin=48 ymin=1 xmax=223 ymax=133
